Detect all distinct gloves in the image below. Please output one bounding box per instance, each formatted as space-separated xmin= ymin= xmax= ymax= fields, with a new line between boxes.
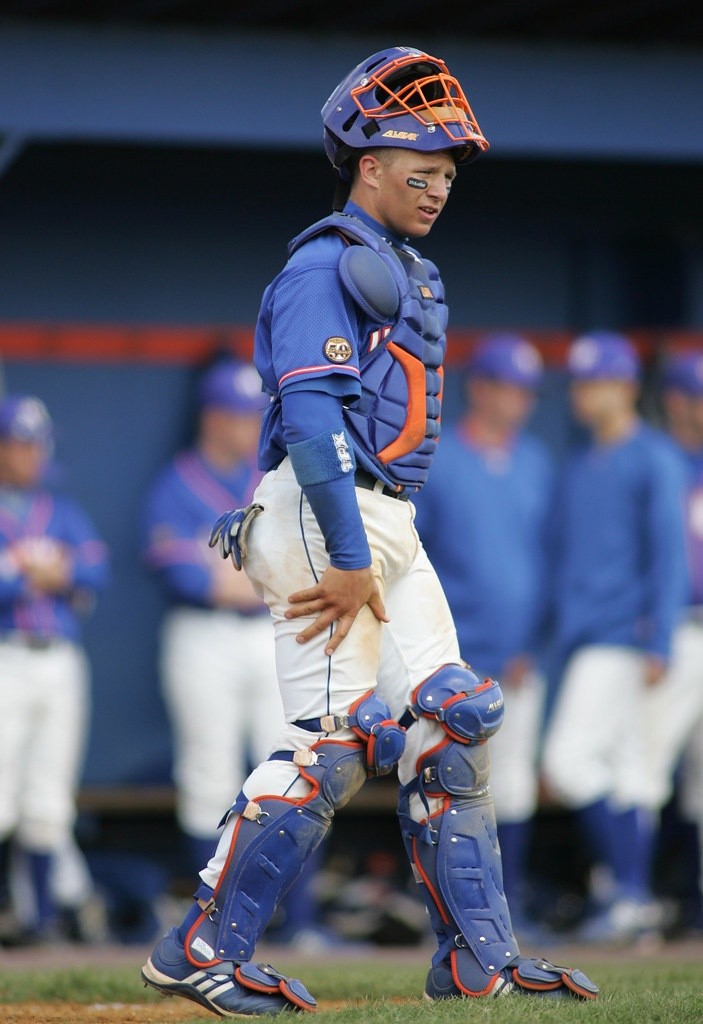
xmin=208 ymin=505 xmax=264 ymax=570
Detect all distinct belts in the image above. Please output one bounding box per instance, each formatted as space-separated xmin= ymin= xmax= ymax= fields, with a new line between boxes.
xmin=353 ymin=467 xmax=409 ymax=502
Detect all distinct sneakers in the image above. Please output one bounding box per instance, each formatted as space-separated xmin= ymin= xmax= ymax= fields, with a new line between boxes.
xmin=423 ymin=957 xmax=576 ymax=1007
xmin=140 ymin=926 xmax=303 ymax=1017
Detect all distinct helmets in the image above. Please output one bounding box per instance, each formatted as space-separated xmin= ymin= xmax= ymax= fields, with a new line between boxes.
xmin=320 ymin=46 xmax=489 ymax=167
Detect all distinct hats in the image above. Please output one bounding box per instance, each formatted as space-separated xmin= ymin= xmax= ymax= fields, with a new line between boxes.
xmin=200 ymin=359 xmax=270 ymax=413
xmin=0 ymin=395 xmax=52 ymax=441
xmin=473 ymin=332 xmax=543 ymax=388
xmin=564 ymin=327 xmax=637 ymax=380
xmin=661 ymin=351 xmax=703 ymax=392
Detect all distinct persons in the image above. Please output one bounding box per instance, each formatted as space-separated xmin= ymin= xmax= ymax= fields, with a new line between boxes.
xmin=1 ymin=386 xmax=111 ymax=950
xmin=140 ymin=46 xmax=602 ymax=1018
xmin=130 ymin=330 xmax=703 ymax=960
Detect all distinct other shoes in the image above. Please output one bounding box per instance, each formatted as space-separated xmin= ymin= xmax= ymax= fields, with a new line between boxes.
xmin=497 ymin=920 xmax=566 ymax=951
xmin=597 ymin=905 xmax=665 ymax=949
